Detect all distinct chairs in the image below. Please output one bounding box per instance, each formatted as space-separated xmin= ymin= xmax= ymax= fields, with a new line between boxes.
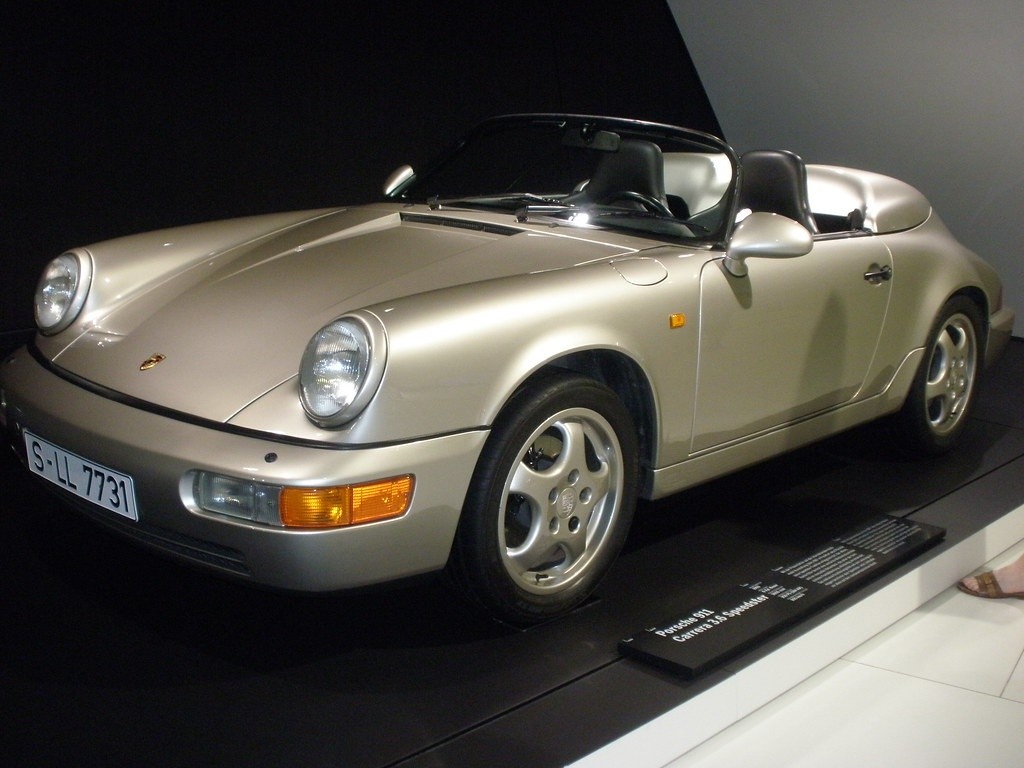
xmin=561 ymin=139 xmax=669 ymax=211
xmin=685 ymin=149 xmax=821 ymax=237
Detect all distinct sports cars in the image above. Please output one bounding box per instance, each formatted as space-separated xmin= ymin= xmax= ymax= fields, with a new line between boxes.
xmin=1 ymin=113 xmax=1014 ymax=631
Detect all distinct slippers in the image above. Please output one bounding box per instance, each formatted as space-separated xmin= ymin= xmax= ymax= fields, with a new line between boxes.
xmin=957 ymin=570 xmax=1024 ymax=599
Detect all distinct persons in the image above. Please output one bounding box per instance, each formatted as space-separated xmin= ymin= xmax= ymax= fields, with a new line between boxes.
xmin=958 ymin=553 xmax=1024 ymax=599
